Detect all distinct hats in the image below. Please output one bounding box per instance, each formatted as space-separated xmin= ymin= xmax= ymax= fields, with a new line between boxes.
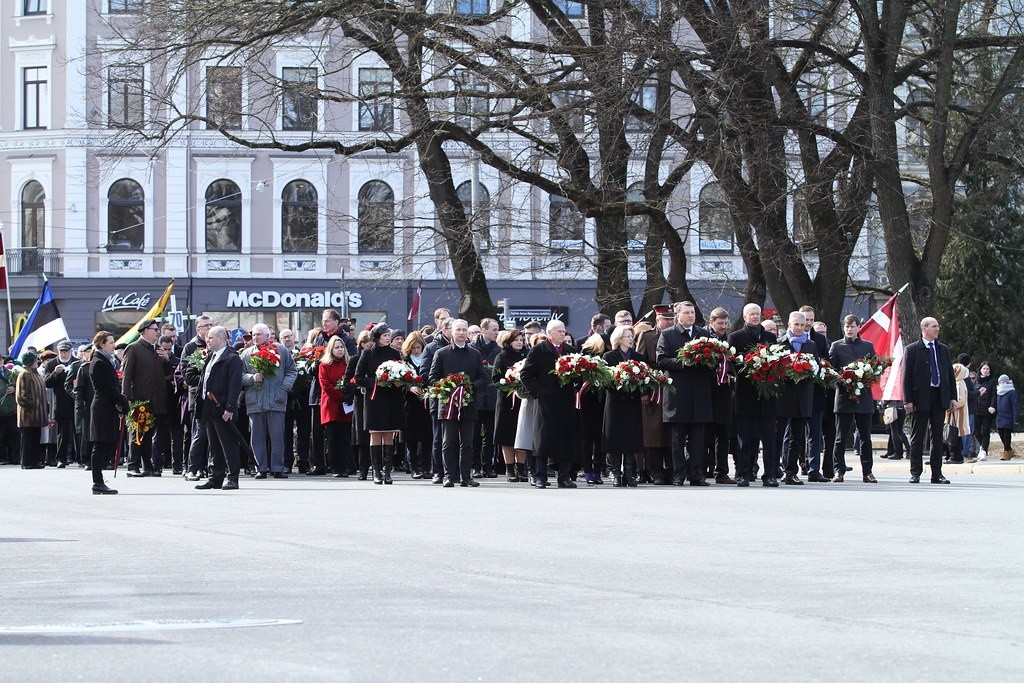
xmin=115 ymin=343 xmax=128 ymax=350
xmin=82 ymin=344 xmax=93 ymax=352
xmin=57 ymin=340 xmax=73 ymax=351
xmin=138 ymin=320 xmax=156 ymax=333
xmin=958 ymin=353 xmax=972 ymax=366
xmin=369 ymin=322 xmax=390 ymax=342
xmin=4 ymin=357 xmax=14 ymax=364
xmin=652 ymin=304 xmax=676 ymax=318
xmin=997 ymin=374 xmax=1010 ymax=384
xmin=39 ymin=350 xmax=58 ymax=363
xmin=243 ymin=335 xmax=253 ymax=343
xmin=391 ymin=329 xmax=405 ymax=343
xmin=22 ymin=352 xmax=37 ymax=368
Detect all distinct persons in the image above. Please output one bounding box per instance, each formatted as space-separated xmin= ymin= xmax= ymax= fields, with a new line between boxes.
xmin=942 ymin=353 xmax=1020 ymax=464
xmin=880 ymin=401 xmax=911 ymax=460
xmin=901 ymin=316 xmax=957 ymax=484
xmin=0 ymin=301 xmax=877 ymax=495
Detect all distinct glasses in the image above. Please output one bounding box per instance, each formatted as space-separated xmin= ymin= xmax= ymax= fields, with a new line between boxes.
xmin=198 ymin=324 xmax=212 ymax=328
xmin=280 ymin=334 xmax=293 ymax=339
xmin=970 ymin=377 xmax=976 ymax=380
xmin=623 ymin=335 xmax=636 ymax=339
xmin=147 ymin=328 xmax=159 ymax=332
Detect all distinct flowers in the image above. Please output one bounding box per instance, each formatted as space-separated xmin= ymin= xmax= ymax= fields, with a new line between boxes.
xmin=375 ymin=359 xmax=423 ymax=395
xmin=291 ymin=346 xmax=325 ymax=373
xmin=483 ymin=357 xmax=529 ymax=399
xmin=248 ymin=341 xmax=282 ymax=389
xmin=184 ymin=346 xmax=209 ymax=371
xmin=126 ymin=399 xmax=155 ymax=446
xmin=420 ymin=372 xmax=475 ymax=407
xmin=548 ymin=336 xmax=895 ymax=400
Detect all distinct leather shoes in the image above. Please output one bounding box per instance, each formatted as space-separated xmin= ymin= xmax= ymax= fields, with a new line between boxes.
xmin=2 ymin=440 xmax=1017 ymax=494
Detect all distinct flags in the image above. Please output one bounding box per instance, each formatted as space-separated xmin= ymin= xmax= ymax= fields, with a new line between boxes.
xmin=859 ymin=293 xmax=904 ymax=400
xmin=115 ymin=283 xmax=173 ymax=345
xmin=10 ymin=282 xmax=66 ymax=362
xmin=0 ymin=233 xmax=7 ymax=292
xmin=407 ymin=281 xmax=421 ymax=324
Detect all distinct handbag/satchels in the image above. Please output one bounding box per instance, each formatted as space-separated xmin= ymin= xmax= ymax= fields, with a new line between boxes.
xmin=882 ymin=408 xmax=898 ymax=425
xmin=943 ymin=411 xmax=958 ymax=446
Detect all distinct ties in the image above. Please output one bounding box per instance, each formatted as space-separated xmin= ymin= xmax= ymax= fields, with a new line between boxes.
xmin=211 ymin=354 xmax=216 ymax=363
xmin=929 ymin=342 xmax=938 ymax=385
xmin=685 ymin=329 xmax=690 ymax=337
